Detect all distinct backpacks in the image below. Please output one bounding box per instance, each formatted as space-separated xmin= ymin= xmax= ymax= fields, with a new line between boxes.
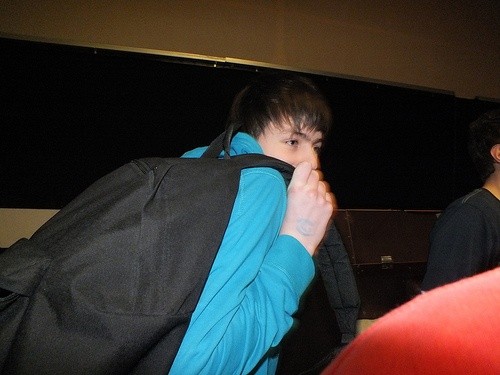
xmin=1 ymin=130 xmax=356 ymax=374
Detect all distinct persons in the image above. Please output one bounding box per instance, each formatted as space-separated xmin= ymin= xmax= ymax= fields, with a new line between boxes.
xmin=3 ymin=73 xmax=334 ymax=375
xmin=421 ymin=136 xmax=500 ymax=287
